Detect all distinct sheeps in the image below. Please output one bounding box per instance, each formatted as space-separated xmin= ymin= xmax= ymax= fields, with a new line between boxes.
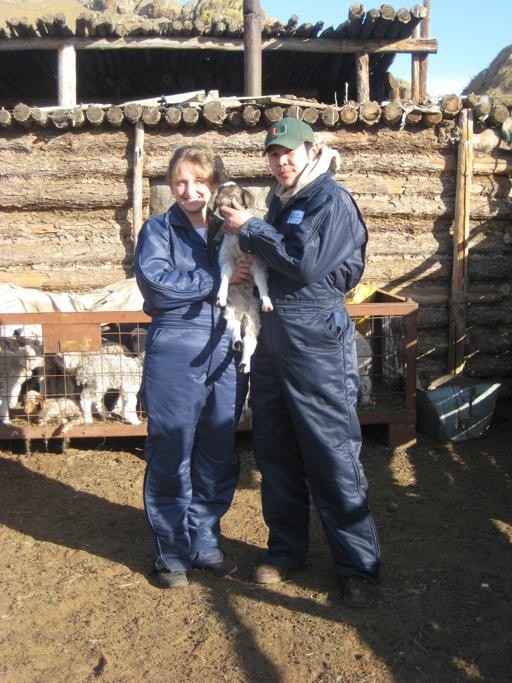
xmin=0 ymin=324 xmax=149 ymax=434
xmin=207 ymin=180 xmax=274 ymax=375
xmin=354 ymin=331 xmax=374 ymax=407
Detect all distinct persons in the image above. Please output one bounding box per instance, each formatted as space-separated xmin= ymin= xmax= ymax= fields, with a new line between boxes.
xmin=219 ymin=117 xmax=383 ymax=610
xmin=135 ymin=143 xmax=249 ymax=589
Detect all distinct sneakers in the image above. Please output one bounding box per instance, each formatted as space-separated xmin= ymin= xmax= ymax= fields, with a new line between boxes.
xmin=202 ymin=553 xmax=239 ymax=577
xmin=155 ymin=567 xmax=189 ymax=590
xmin=249 ymin=561 xmax=294 ymax=584
xmin=342 ymin=577 xmax=378 ymax=609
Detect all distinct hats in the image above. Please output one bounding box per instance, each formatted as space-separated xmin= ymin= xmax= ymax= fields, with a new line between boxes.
xmin=262 ymin=117 xmax=315 ymax=157
